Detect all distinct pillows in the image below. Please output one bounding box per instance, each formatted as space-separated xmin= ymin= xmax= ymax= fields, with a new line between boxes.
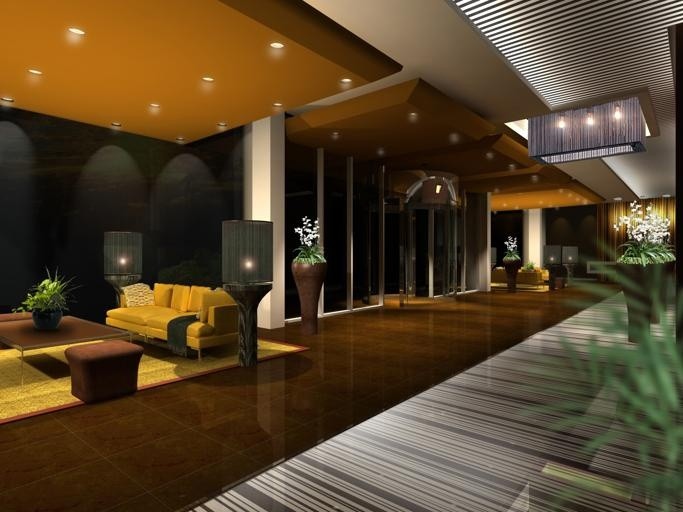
xmin=120 ymin=283 xmax=236 ymax=323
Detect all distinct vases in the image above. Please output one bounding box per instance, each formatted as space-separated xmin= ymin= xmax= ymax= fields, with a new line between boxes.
xmin=292 ymin=258 xmax=326 ymax=335
xmin=616 ymin=253 xmax=676 ymax=344
xmin=503 ymin=255 xmax=521 ymax=293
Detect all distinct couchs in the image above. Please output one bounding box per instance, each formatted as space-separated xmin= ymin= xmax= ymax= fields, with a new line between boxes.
xmin=106 ymin=294 xmax=239 ymax=362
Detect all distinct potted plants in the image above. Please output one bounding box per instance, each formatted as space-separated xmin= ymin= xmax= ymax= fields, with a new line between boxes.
xmin=12 ymin=267 xmax=81 ymax=330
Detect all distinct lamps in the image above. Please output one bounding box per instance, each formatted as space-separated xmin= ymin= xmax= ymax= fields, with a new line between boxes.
xmin=528 ymin=97 xmax=647 ymax=165
xmin=104 ymin=231 xmax=143 ymax=305
xmin=222 ymin=220 xmax=273 ymax=367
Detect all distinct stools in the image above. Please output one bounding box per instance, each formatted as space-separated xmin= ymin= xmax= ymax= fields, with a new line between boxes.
xmin=65 ymin=340 xmax=144 ymax=404
xmin=0 ymin=312 xmax=32 ymax=349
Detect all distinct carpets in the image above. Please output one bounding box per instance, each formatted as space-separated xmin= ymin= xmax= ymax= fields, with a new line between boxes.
xmin=0 ymin=338 xmax=311 ymax=425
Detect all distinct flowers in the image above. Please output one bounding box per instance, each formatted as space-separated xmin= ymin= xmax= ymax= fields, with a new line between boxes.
xmin=504 ymin=236 xmax=520 ymax=261
xmin=612 ymin=201 xmax=676 ymax=267
xmin=292 ymin=215 xmax=326 ymax=266
